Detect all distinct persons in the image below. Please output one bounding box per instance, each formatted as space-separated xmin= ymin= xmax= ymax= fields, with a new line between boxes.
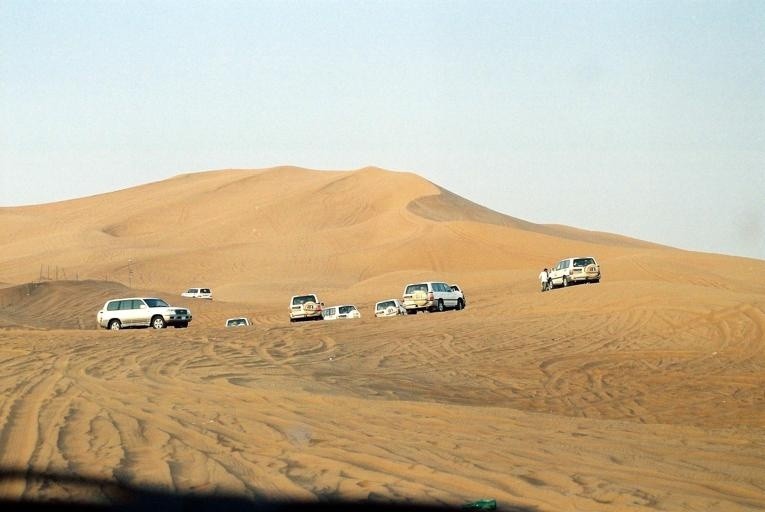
xmin=537 ymin=267 xmax=551 ymax=292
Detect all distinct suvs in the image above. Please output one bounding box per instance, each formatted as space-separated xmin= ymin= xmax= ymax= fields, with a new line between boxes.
xmin=288 ymin=294 xmax=324 ymax=320
xmin=225 ymin=317 xmax=252 ymax=327
xmin=374 ymin=281 xmax=465 ymax=318
xmin=548 ymin=256 xmax=601 ymax=289
xmin=322 ymin=305 xmax=360 ymax=321
xmin=96 ymin=296 xmax=192 ymax=330
xmin=181 ymin=287 xmax=212 ymax=301
